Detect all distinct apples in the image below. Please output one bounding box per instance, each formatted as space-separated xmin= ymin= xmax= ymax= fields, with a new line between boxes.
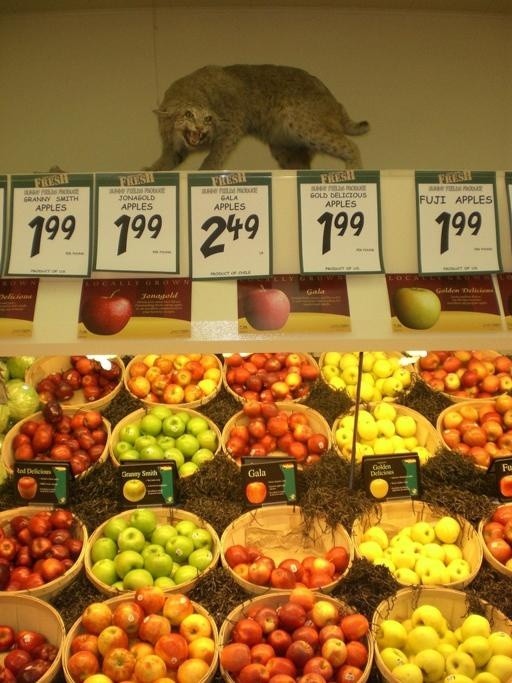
xmin=246 ymin=481 xmax=267 ymax=504
xmin=128 ymin=353 xmax=221 ymax=404
xmin=335 ymin=402 xmax=429 ymax=464
xmin=358 ymin=518 xmax=471 ymax=587
xmin=113 ymin=406 xmax=219 ymax=478
xmin=323 ymin=352 xmax=412 ymax=402
xmin=483 ymin=505 xmax=511 ymax=571
xmin=35 ymin=355 xmax=121 ymax=404
xmin=81 ymin=288 xmax=131 ymax=337
xmin=226 ymin=401 xmax=328 ymax=466
xmin=225 ymin=544 xmax=350 ymax=589
xmin=91 ymin=509 xmax=210 ymax=593
xmin=123 ymin=480 xmax=146 ymax=502
xmin=377 ymin=605 xmax=512 ymax=683
xmin=370 ymin=479 xmax=387 ymax=498
xmin=242 ymin=283 xmax=290 ymax=330
xmin=501 ymin=474 xmax=512 ymax=496
xmin=67 ymin=584 xmax=215 ymax=683
xmin=392 ymin=286 xmax=442 ymax=330
xmin=442 ymin=395 xmax=511 ymax=466
xmin=0 ymin=625 xmax=57 ymax=683
xmin=17 ymin=477 xmax=37 ymax=500
xmin=420 ymin=351 xmax=511 ymax=399
xmin=11 ymin=402 xmax=108 ymax=474
xmin=225 ymin=352 xmax=319 ymax=405
xmin=220 ymin=587 xmax=370 ymax=683
xmin=1 ymin=509 xmax=82 ymax=590
